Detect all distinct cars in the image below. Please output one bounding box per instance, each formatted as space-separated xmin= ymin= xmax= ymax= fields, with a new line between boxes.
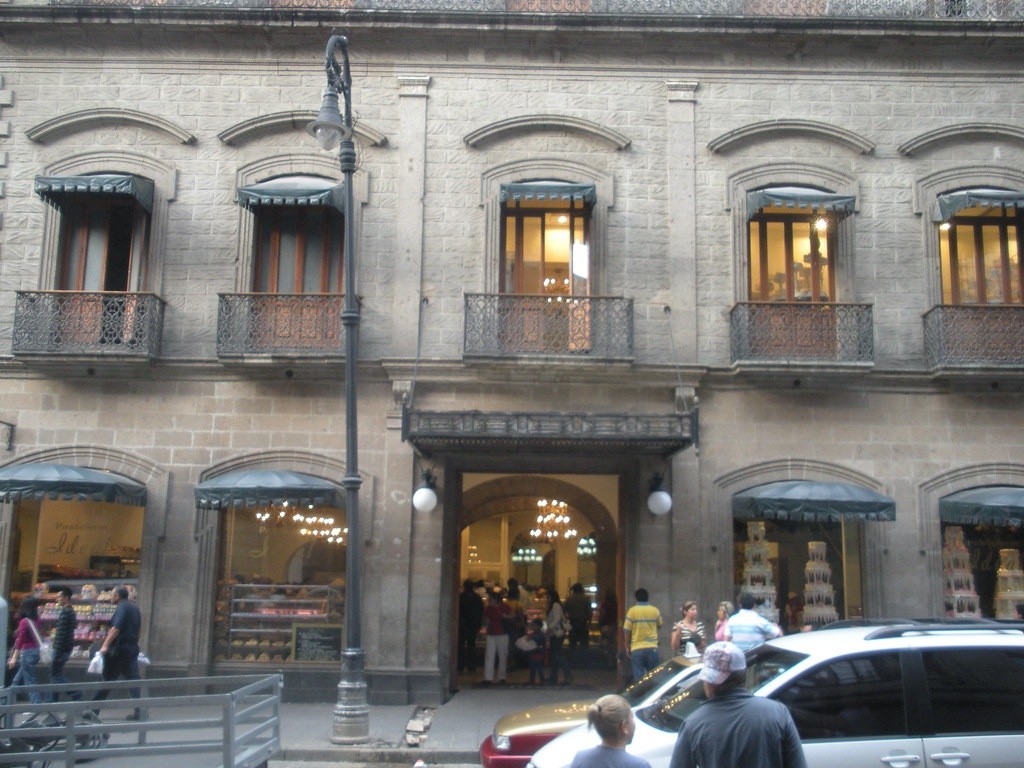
xmin=478 ymin=654 xmax=704 ymax=768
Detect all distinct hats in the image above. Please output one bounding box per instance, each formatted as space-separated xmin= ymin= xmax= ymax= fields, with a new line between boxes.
xmin=699 ymin=641 xmax=747 ymax=685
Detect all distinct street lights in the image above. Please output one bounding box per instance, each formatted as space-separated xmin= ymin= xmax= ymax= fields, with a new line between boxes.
xmin=307 ymin=34 xmax=375 ymax=747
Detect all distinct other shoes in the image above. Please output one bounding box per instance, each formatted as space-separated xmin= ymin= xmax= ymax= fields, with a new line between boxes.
xmin=126 ymin=710 xmax=149 ymax=721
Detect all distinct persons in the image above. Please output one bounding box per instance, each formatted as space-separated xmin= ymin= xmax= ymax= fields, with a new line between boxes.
xmin=671 ymin=601 xmax=706 ymax=657
xmin=7 ymin=595 xmax=44 ymax=715
xmin=459 ymin=577 xmax=618 ymax=686
xmin=715 ymin=592 xmax=813 ymax=689
xmin=45 ymin=586 xmax=83 ymax=703
xmin=623 ymin=588 xmax=663 ymax=682
xmin=83 ymin=586 xmax=141 ymax=720
xmin=669 ymin=641 xmax=808 ymax=768
xmin=233 ymin=568 xmax=345 ymax=610
xmin=7 ymin=610 xmax=20 ymax=649
xmin=788 ymin=660 xmax=900 ymax=739
xmin=570 ymin=695 xmax=653 ymax=768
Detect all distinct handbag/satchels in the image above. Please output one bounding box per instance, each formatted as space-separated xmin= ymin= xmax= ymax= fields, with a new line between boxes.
xmin=39 ymin=645 xmax=51 ymax=664
xmin=553 ymin=619 xmax=572 ymax=638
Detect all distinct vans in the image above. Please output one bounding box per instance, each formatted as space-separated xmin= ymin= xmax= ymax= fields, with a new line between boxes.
xmin=525 ymin=617 xmax=1024 ymax=768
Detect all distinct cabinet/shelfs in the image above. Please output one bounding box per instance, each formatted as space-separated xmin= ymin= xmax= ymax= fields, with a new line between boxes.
xmin=227 ymin=583 xmax=333 ymax=660
xmin=36 ymin=597 xmax=117 ymax=645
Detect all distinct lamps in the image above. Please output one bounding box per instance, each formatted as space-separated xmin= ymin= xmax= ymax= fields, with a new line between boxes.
xmin=529 ymin=498 xmax=578 ymax=546
xmin=411 ymin=467 xmax=438 ymax=513
xmin=646 ymin=470 xmax=672 ymax=519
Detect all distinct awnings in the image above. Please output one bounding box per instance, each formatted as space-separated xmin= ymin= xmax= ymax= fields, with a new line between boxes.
xmin=194 ymin=469 xmax=345 ymax=510
xmin=234 ymin=176 xmax=344 ymax=213
xmin=499 ymin=181 xmax=597 ymax=206
xmin=34 ymin=175 xmax=154 ymax=215
xmin=733 ymin=481 xmax=896 ymax=523
xmin=0 ymin=464 xmax=146 ymax=507
xmin=940 ymin=487 xmax=1024 ymax=527
xmin=938 ymin=189 xmax=1024 ymax=222
xmin=746 ymin=187 xmax=856 ymax=224
